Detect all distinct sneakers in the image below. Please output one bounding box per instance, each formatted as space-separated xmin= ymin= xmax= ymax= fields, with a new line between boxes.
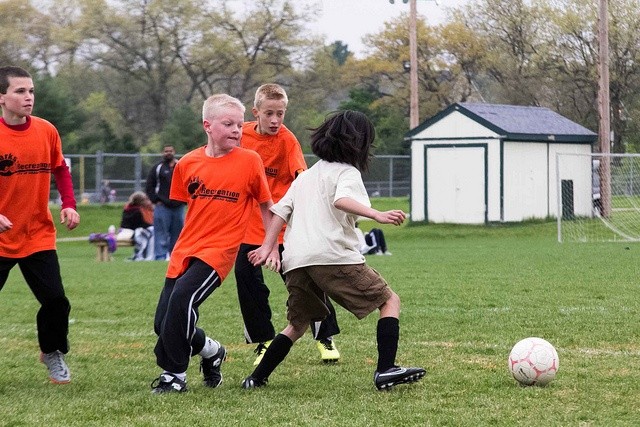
xmin=200 ymin=340 xmax=227 ymax=389
xmin=317 ymin=339 xmax=340 ymax=363
xmin=374 ymin=366 xmax=426 ymax=390
xmin=241 ymin=376 xmax=268 ymax=389
xmin=39 ymin=351 xmax=71 ymax=384
xmin=253 ymin=339 xmax=272 ymax=367
xmin=149 ymin=373 xmax=189 ymax=397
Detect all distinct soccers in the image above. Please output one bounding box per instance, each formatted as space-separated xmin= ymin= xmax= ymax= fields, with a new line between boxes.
xmin=508 ymin=338 xmax=559 ymax=386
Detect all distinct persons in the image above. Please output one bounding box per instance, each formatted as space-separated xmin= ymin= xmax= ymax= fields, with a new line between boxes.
xmin=146 ymin=145 xmax=185 ymax=260
xmin=239 ymin=84 xmax=340 ymax=369
xmin=364 ymin=228 xmax=392 ymax=256
xmin=151 ymin=91 xmax=281 ymax=395
xmin=120 ymin=192 xmax=154 ymax=260
xmin=0 ymin=66 xmax=80 ymax=386
xmin=242 ymin=108 xmax=426 ymax=391
xmin=101 ymin=180 xmax=112 ymax=202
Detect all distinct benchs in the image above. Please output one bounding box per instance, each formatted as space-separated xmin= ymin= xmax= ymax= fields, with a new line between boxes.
xmin=89 ymin=231 xmax=138 ymax=263
